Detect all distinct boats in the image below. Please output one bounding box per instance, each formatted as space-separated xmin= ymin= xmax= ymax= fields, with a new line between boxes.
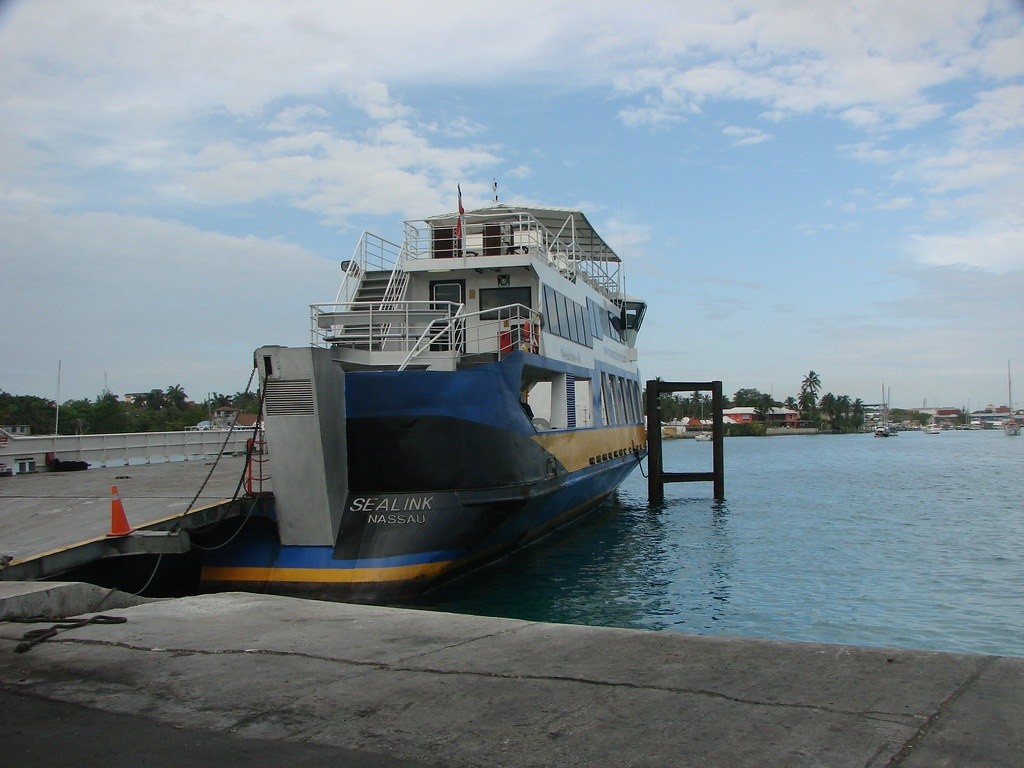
xmin=695 ymin=431 xmax=713 ymax=441
xmin=200 ymin=176 xmax=648 ymax=605
xmin=922 ymin=423 xmax=940 ymax=434
xmin=1001 ymin=418 xmax=1020 ymax=436
xmin=875 ymin=425 xmax=890 ymax=438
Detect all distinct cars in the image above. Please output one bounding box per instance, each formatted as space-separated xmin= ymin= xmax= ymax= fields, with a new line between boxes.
xmin=198 ymin=420 xmax=212 ymax=430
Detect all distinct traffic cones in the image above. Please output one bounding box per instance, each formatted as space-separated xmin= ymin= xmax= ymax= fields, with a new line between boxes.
xmin=105 ymin=485 xmax=136 ymax=536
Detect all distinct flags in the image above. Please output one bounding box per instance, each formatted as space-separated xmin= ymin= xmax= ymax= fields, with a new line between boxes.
xmin=455 ymin=200 xmax=464 ymax=237
